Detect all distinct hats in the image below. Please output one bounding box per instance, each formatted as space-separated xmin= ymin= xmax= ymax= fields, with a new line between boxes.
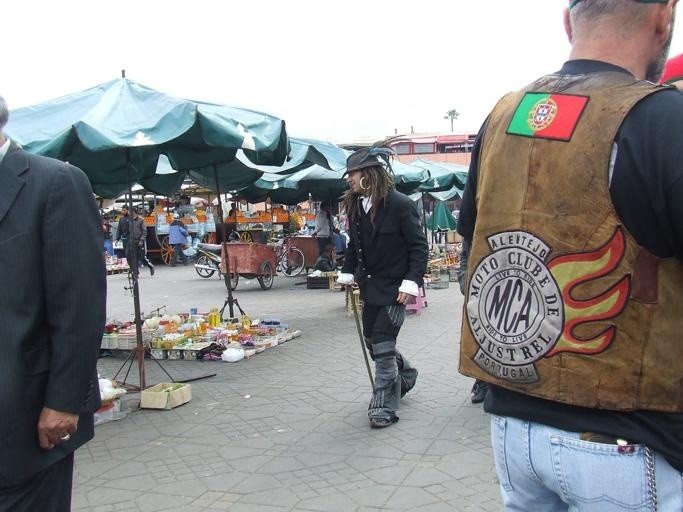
xmin=341 ymin=146 xmax=385 ymax=180
xmin=173 ymin=214 xmax=180 ymax=219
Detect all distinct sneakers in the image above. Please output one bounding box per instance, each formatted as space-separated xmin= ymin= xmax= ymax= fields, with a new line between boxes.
xmin=369 ymin=368 xmax=418 ymax=427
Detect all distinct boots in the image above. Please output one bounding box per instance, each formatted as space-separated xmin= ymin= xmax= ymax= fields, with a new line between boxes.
xmin=471 ymin=378 xmax=488 ymax=404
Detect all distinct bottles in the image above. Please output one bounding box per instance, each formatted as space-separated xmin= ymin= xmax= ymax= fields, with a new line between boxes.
xmin=232 ymin=332 xmax=239 ymax=342
xmin=152 ymin=331 xmax=162 ymax=349
xmin=165 ymin=320 xmax=178 ymax=333
xmin=241 ymin=313 xmax=250 ymax=333
xmin=208 ymin=307 xmax=220 ymax=328
xmin=128 ymin=269 xmax=135 ymax=288
xmin=446 ymin=254 xmax=455 ymax=265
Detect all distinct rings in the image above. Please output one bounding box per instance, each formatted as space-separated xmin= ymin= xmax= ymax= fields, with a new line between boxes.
xmin=60 ymin=433 xmax=71 ymax=441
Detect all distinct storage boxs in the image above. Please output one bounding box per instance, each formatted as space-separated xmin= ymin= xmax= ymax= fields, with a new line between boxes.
xmin=307 ymin=272 xmax=334 ymax=289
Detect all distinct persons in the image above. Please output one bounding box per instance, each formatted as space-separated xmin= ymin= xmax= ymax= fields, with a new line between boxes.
xmin=337 ymin=141 xmax=429 ymax=428
xmin=456 ymin=0 xmax=683 ymax=512
xmin=0 ymin=95 xmax=106 ymax=512
xmin=167 ymin=214 xmax=189 ymax=266
xmin=115 ymin=205 xmax=155 ymax=276
xmin=313 ymin=202 xmax=337 ymax=271
xmin=225 ymin=203 xmax=236 ymax=223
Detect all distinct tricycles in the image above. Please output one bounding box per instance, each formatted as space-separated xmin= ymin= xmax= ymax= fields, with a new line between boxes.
xmin=194 ymin=229 xmax=339 ymax=290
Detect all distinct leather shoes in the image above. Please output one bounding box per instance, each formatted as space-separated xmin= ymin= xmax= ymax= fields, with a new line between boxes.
xmin=148 ymin=262 xmax=154 ymax=276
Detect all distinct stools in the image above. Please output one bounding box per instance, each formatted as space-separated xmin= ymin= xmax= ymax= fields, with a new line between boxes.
xmin=346 ymin=289 xmax=366 ymax=318
xmin=403 ymin=280 xmax=429 ymax=316
